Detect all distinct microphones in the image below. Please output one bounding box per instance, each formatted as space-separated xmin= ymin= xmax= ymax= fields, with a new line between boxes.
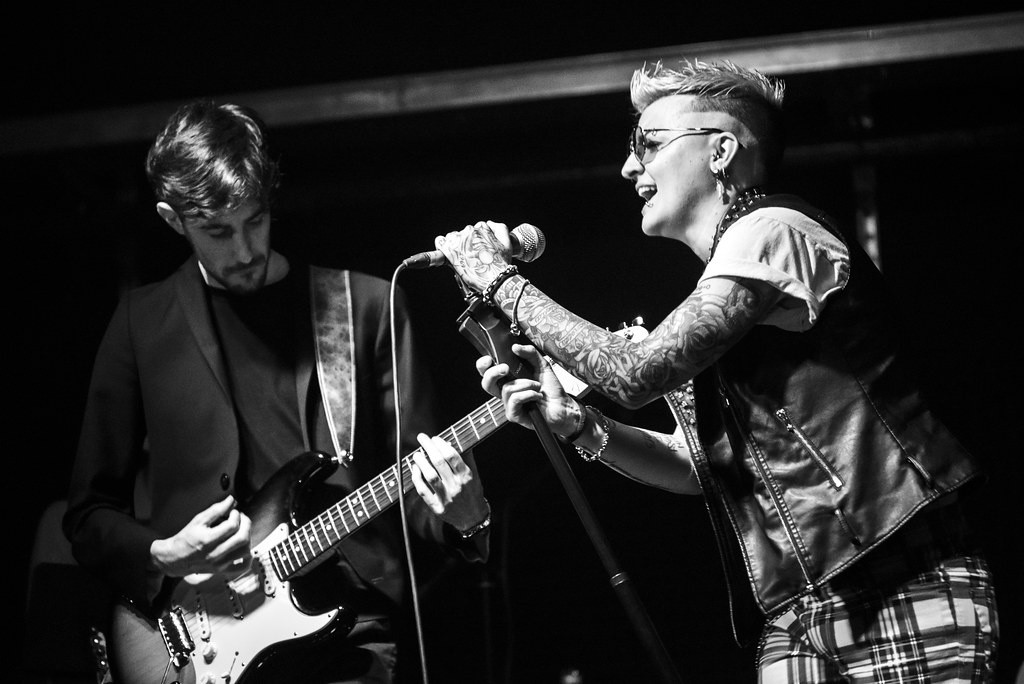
xmin=402 ymin=223 xmax=547 ymax=269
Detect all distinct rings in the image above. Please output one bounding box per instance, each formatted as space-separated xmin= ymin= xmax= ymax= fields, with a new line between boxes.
xmin=232 ymin=557 xmax=243 ymax=566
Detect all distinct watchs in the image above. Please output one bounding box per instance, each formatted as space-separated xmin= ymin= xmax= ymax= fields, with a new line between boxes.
xmin=458 ymin=497 xmax=491 ymax=540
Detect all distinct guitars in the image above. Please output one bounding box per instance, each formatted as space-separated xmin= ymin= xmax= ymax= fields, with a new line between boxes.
xmin=103 ymin=310 xmax=650 ymax=684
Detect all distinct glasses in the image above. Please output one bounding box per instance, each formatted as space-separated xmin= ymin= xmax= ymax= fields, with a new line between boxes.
xmin=627 ymin=126 xmax=744 ymax=162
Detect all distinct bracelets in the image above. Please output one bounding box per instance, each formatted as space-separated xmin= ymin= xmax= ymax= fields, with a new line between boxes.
xmin=557 ymin=393 xmax=587 ymax=445
xmin=483 ymin=265 xmax=519 ymax=306
xmin=575 ymin=404 xmax=610 ymax=461
xmin=510 ymin=279 xmax=530 ymax=335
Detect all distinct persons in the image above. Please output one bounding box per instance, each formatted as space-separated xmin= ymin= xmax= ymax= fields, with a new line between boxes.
xmin=436 ymin=61 xmax=999 ymax=684
xmin=64 ymin=102 xmax=505 ymax=683
